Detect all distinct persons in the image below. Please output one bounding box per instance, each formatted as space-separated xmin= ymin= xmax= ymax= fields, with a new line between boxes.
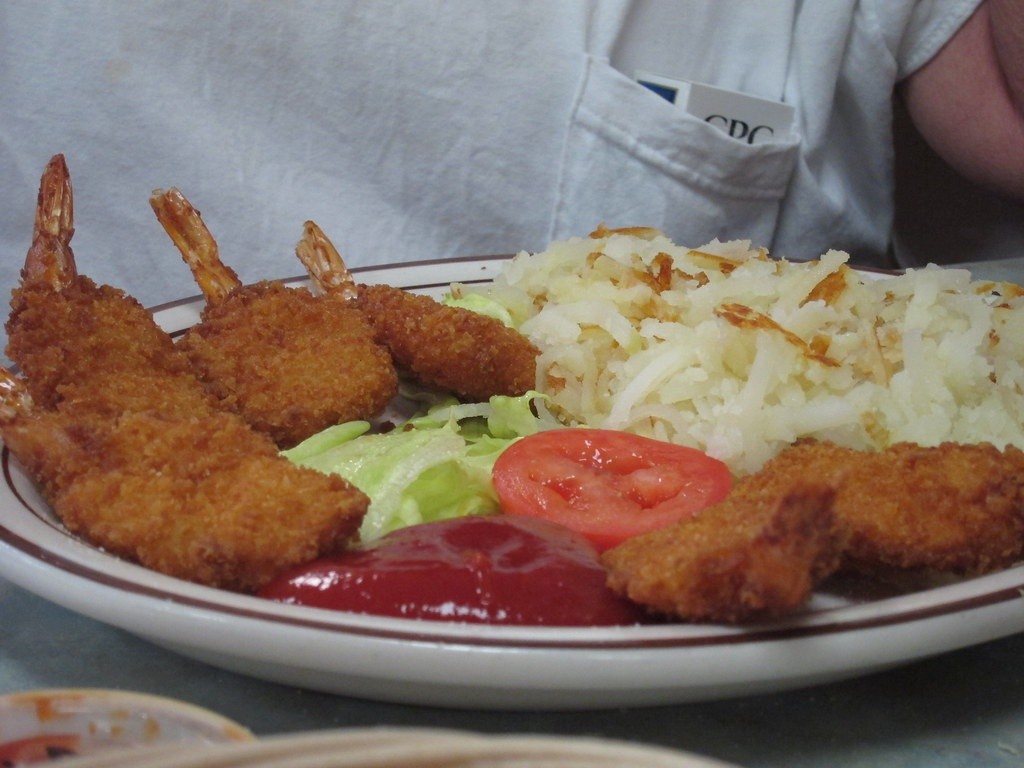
xmin=0 ymin=0 xmax=1024 ymax=370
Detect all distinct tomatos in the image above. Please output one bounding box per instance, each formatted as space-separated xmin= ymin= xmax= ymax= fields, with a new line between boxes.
xmin=490 ymin=430 xmax=734 ymax=557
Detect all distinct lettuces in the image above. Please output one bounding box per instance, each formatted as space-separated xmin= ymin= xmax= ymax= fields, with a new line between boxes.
xmin=273 ymin=292 xmax=553 ymax=545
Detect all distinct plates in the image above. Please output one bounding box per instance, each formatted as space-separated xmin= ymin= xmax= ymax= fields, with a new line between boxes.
xmin=1 ymin=257 xmax=1024 ymax=711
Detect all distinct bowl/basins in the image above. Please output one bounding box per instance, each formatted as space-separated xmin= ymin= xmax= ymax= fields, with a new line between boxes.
xmin=0 ymin=691 xmax=256 ymax=768
